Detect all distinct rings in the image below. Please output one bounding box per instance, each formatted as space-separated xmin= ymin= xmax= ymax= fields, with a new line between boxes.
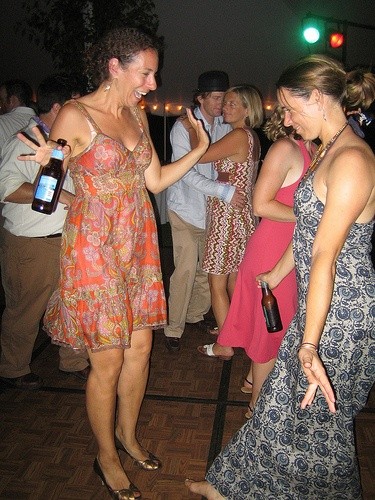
xmin=181 ymin=117 xmax=183 ymax=120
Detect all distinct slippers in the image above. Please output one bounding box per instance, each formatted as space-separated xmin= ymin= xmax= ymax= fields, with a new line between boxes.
xmin=208 ymin=326 xmax=220 ymax=336
xmin=197 ymin=343 xmax=231 ymax=361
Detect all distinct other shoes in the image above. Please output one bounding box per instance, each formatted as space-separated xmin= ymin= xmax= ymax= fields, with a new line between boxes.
xmin=71 ymin=367 xmax=91 ymax=379
xmin=165 ymin=336 xmax=180 ymax=351
xmin=0 ymin=373 xmax=42 ymax=390
xmin=189 ymin=320 xmax=211 ymax=333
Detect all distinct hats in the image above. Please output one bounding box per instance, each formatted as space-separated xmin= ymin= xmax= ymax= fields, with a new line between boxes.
xmin=192 ymin=70 xmax=230 ymax=93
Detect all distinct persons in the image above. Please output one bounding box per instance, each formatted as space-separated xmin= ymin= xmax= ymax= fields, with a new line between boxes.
xmin=0 ymin=74 xmax=91 ymax=389
xmin=0 ymin=80 xmax=36 ymax=150
xmin=217 ymin=97 xmax=316 ymax=420
xmin=162 ymin=70 xmax=248 ymax=352
xmin=175 ymin=84 xmax=264 ymax=359
xmin=182 ymin=54 xmax=375 ymax=500
xmin=17 ymin=27 xmax=209 ymax=500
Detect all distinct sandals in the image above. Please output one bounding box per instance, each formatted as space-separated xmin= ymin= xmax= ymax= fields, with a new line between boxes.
xmin=240 ymin=377 xmax=252 ymax=394
xmin=245 ymin=404 xmax=254 ymax=419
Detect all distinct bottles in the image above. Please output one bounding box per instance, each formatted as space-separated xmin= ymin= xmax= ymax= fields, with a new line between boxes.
xmin=31 ymin=139 xmax=67 ymax=215
xmin=259 ymin=280 xmax=284 ymax=333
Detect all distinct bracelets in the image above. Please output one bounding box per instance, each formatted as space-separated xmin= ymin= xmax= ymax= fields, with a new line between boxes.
xmin=300 ymin=343 xmax=318 ymax=352
xmin=220 ymin=184 xmax=231 ymax=201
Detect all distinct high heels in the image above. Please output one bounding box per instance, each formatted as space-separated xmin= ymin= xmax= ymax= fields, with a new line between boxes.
xmin=113 ymin=430 xmax=161 ymax=472
xmin=92 ymin=456 xmax=142 ymax=500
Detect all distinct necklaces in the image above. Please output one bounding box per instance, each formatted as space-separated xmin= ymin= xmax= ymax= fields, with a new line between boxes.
xmin=299 ymin=121 xmax=350 ymax=186
